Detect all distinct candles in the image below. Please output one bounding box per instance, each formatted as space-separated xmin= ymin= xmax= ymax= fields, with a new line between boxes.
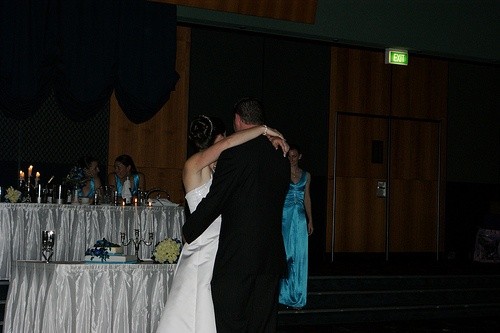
xmin=20 ymin=165 xmax=40 ymax=181
xmin=38 ymin=184 xmax=41 ymax=198
xmin=59 ymin=184 xmax=61 ymax=198
xmin=149 ymin=201 xmax=152 ymax=233
xmin=122 ymin=202 xmax=125 ymax=233
xmin=135 ymin=199 xmax=138 ymax=229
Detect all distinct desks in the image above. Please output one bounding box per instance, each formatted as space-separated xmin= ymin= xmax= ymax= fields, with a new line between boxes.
xmin=3 ymin=260 xmax=178 ymax=333
xmin=0 ymin=202 xmax=186 ymax=302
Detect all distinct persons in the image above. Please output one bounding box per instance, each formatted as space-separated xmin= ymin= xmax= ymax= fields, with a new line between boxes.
xmin=182 ymin=97 xmax=291 ymax=333
xmin=108 ymin=154 xmax=145 ymax=195
xmin=80 ymin=158 xmax=101 ymax=199
xmin=279 ymin=148 xmax=313 ymax=310
xmin=155 ymin=115 xmax=289 ymax=333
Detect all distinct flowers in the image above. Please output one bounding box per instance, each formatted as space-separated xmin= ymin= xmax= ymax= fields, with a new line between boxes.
xmin=6 ymin=186 xmax=22 ymax=202
xmin=152 ymin=237 xmax=182 ymax=264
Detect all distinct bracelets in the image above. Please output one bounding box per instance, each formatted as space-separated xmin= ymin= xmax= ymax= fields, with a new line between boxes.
xmin=262 ymin=125 xmax=267 ymax=135
